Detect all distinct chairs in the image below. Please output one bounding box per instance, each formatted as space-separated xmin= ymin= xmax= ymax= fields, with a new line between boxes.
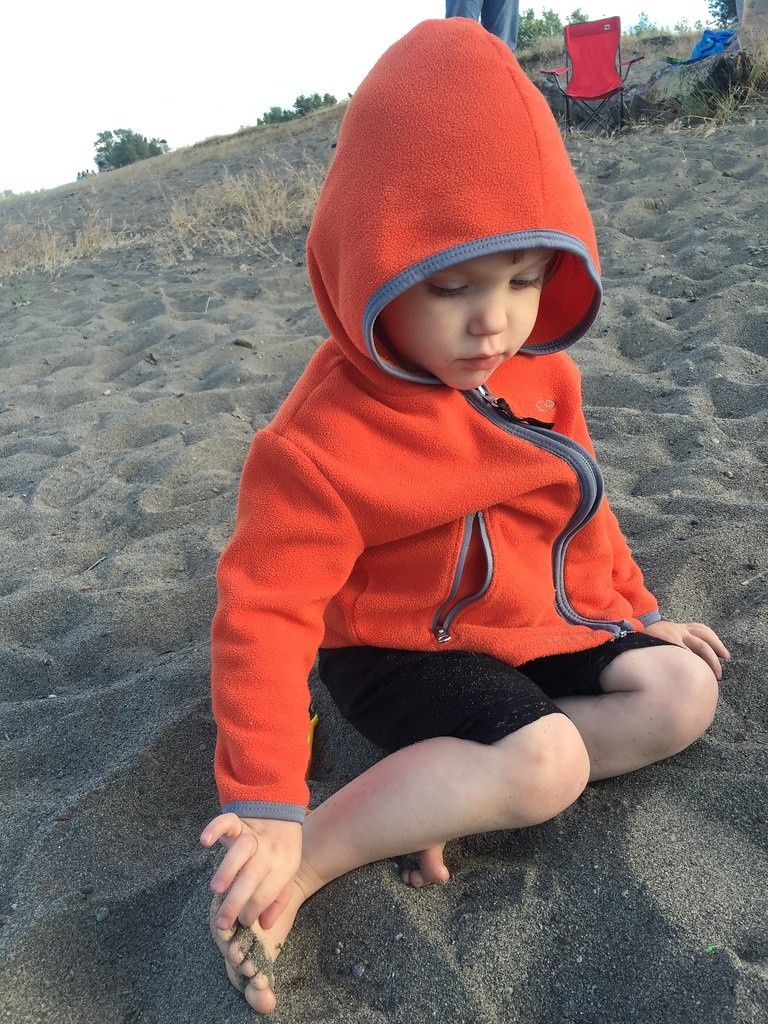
xmin=540 ymin=16 xmax=645 ymax=133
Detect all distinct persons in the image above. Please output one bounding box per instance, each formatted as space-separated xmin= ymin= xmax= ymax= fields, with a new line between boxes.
xmin=446 ymin=0 xmax=519 ymax=51
xmin=199 ymin=18 xmax=731 ymax=1009
